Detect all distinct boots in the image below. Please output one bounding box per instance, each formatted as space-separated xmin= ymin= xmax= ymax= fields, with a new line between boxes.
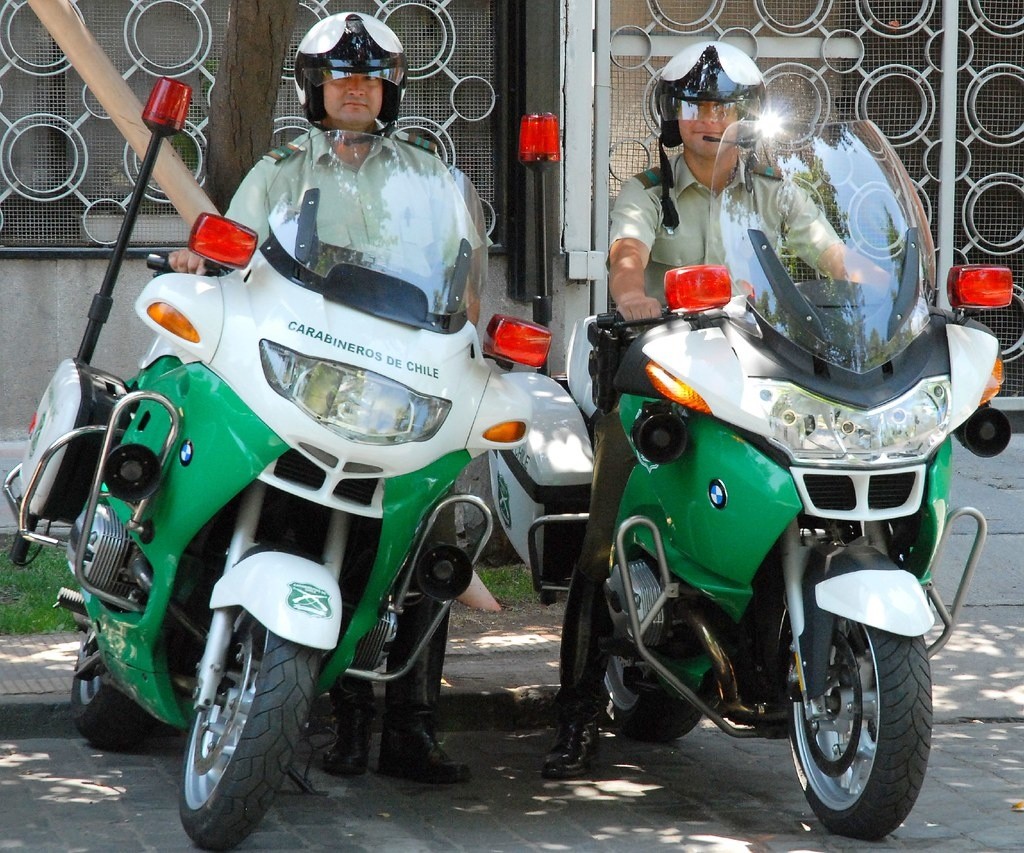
xmin=541 ymin=564 xmax=614 ymax=780
xmin=322 ymin=674 xmax=376 ymax=775
xmin=378 ymin=590 xmax=477 ymax=784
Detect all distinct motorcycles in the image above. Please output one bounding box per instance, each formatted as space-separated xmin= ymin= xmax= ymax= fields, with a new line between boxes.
xmin=1 ymin=118 xmax=552 ymax=853
xmin=486 ymin=118 xmax=1013 ymax=843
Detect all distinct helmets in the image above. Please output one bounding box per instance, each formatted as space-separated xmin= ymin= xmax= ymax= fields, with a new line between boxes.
xmin=294 ymin=12 xmax=408 ymax=122
xmin=655 ymin=41 xmax=767 ymax=148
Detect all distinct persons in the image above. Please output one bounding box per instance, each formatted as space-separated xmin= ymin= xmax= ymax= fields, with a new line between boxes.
xmin=166 ymin=10 xmax=894 ymax=784
